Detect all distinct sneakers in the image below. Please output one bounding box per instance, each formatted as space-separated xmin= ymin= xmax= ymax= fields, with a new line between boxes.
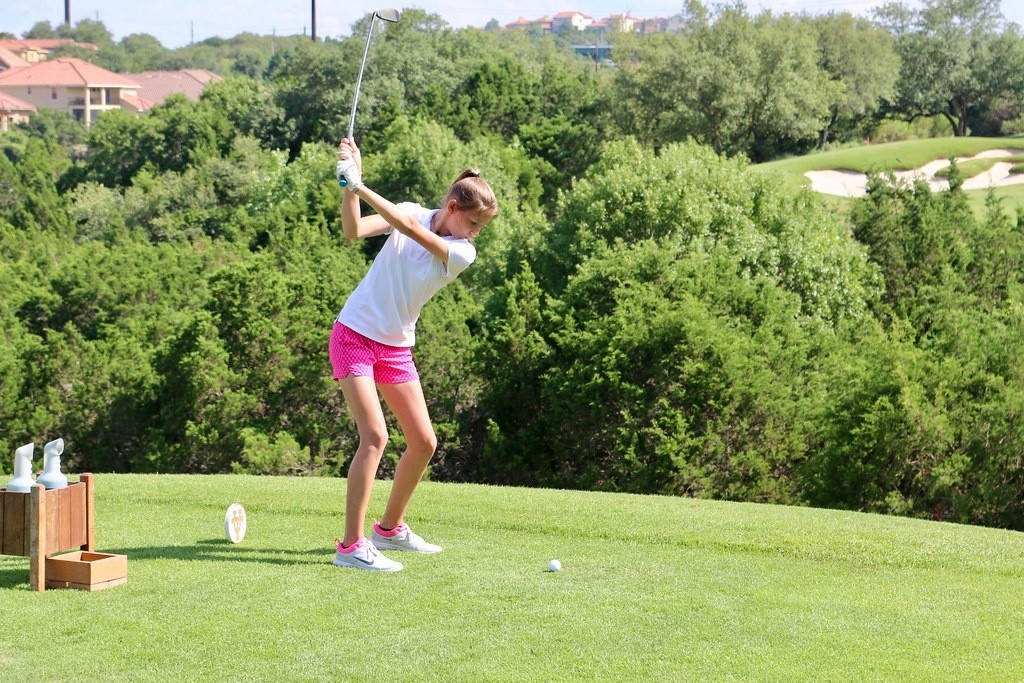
xmin=333 ymin=536 xmax=403 ymax=572
xmin=370 ymin=518 xmax=442 ymax=554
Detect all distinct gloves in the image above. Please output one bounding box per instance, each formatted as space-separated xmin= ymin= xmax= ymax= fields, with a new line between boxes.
xmin=336 ymin=151 xmax=364 ymax=192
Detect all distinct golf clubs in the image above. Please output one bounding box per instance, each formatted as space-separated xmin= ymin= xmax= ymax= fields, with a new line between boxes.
xmin=338 ymin=8 xmax=402 ymax=187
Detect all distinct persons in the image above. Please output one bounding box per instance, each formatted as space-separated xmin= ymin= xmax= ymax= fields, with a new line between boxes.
xmin=330 ymin=137 xmax=498 ymax=574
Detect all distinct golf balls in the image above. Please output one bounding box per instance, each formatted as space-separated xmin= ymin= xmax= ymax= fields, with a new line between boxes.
xmin=549 ymin=560 xmax=563 ymax=573
